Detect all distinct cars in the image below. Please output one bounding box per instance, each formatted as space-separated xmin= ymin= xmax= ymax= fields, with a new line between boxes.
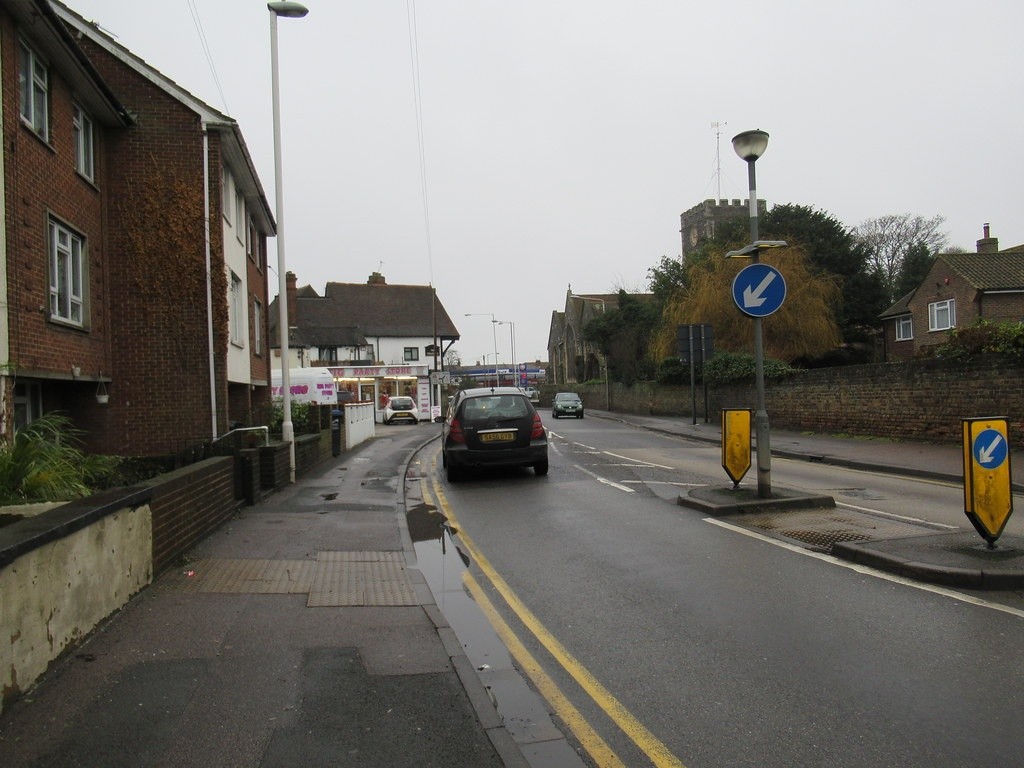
xmin=384 ymin=396 xmax=419 ymax=425
xmin=552 ymin=393 xmax=585 ymax=419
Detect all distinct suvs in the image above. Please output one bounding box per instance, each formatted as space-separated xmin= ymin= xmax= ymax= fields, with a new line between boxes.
xmin=434 ymin=386 xmax=549 ymax=481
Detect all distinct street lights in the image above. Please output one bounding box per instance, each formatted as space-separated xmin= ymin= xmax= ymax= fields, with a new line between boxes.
xmin=491 ymin=319 xmax=517 ymax=387
xmin=487 ymin=352 xmax=500 ymax=386
xmin=465 ymin=312 xmax=500 ymax=387
xmin=268 ymin=1 xmax=309 ymax=480
xmin=731 ymin=126 xmax=777 ymax=498
